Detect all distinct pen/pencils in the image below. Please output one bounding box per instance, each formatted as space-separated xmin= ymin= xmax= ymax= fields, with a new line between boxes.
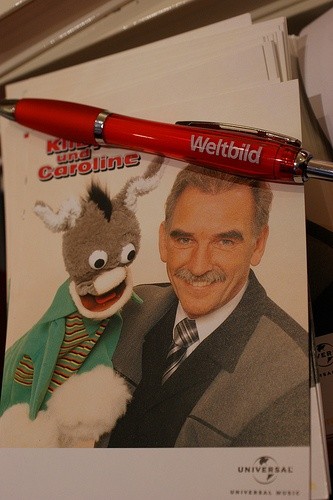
xmin=0 ymin=97 xmax=333 ymax=185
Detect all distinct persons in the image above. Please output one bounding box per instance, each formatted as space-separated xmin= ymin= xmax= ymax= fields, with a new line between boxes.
xmin=95 ymin=165 xmax=310 ymax=447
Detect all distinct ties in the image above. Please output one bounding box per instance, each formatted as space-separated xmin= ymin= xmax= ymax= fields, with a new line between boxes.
xmin=159 ymin=316 xmax=200 ymax=387
